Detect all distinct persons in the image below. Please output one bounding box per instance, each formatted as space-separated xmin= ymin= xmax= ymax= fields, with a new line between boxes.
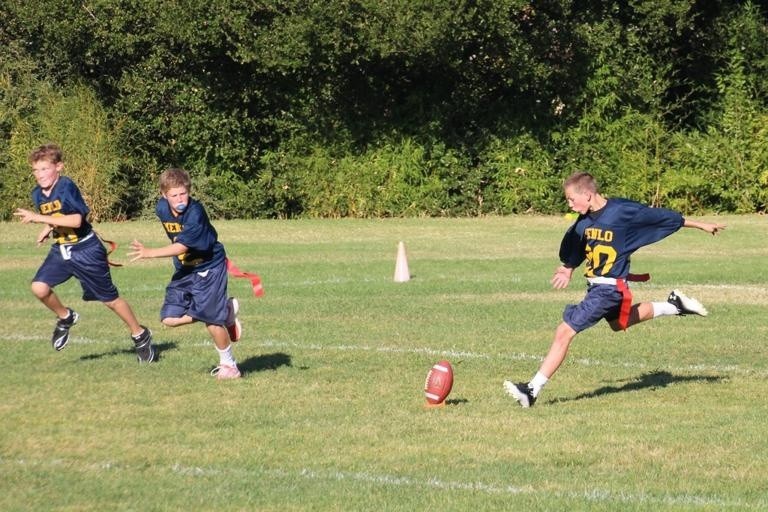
xmin=13 ymin=145 xmax=155 ymax=366
xmin=126 ymin=167 xmax=242 ymax=383
xmin=502 ymin=172 xmax=727 ymax=410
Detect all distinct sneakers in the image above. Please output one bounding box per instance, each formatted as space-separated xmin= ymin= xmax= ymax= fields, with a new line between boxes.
xmin=212 ymin=363 xmax=240 ymax=379
xmin=51 ymin=308 xmax=78 ymax=351
xmin=226 ymin=297 xmax=241 ymax=342
xmin=131 ymin=326 xmax=154 ymax=363
xmin=668 ymin=289 xmax=708 ymax=318
xmin=504 ymin=380 xmax=536 ymax=407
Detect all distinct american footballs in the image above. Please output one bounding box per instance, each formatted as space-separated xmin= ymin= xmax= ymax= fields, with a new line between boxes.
xmin=424 ymin=360 xmax=453 ymax=405
